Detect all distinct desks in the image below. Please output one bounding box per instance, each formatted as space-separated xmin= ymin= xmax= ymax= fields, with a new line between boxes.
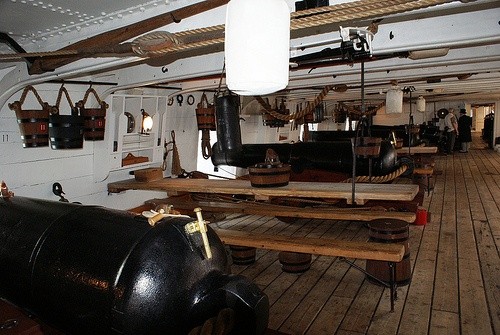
xmin=110 ymin=175 xmax=420 ymax=204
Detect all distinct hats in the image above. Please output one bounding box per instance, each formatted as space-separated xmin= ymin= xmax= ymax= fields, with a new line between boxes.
xmin=459 ymin=109 xmax=466 ymax=114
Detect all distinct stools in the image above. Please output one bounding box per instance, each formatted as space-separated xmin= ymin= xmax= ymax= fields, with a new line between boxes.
xmin=414 ymin=166 xmax=432 ymax=195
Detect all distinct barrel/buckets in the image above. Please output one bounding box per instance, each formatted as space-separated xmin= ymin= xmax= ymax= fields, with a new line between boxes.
xmin=395 ymin=139 xmax=403 ymax=148
xmin=350 ymin=136 xmax=382 ymax=159
xmin=195 ymin=95 xmax=215 ymax=130
xmin=249 ymin=162 xmax=291 ymax=187
xmin=260 ymin=102 xmax=368 ymax=127
xmin=77 ymin=88 xmax=109 ymax=141
xmin=277 ymin=251 xmax=311 ymax=272
xmin=46 ymin=87 xmax=84 ymax=150
xmin=365 ymin=217 xmax=412 ymax=285
xmin=9 ymin=85 xmax=52 ymax=148
xmin=229 ymin=244 xmax=256 ymax=265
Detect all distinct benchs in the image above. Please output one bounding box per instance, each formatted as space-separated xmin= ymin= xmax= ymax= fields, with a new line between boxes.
xmin=215 ymin=228 xmax=406 ymax=313
xmin=144 ymin=198 xmax=416 ymax=225
xmin=398 ymin=146 xmax=438 ymax=152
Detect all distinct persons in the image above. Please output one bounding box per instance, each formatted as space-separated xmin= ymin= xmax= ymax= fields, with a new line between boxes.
xmin=458 ymin=108 xmax=472 ymax=153
xmin=444 ymin=107 xmax=458 ymax=155
xmin=483 ymin=115 xmax=494 ymax=149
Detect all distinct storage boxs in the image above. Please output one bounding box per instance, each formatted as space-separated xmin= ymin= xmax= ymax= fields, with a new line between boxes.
xmin=135 ymin=168 xmax=164 ymax=181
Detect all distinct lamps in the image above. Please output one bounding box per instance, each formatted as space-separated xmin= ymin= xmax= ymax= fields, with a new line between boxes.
xmin=416 ymin=95 xmax=424 ymax=112
xmin=224 ymin=0 xmax=290 ymax=96
xmin=386 ymin=81 xmax=403 ymax=114
xmin=408 ymin=47 xmax=450 ymax=59
